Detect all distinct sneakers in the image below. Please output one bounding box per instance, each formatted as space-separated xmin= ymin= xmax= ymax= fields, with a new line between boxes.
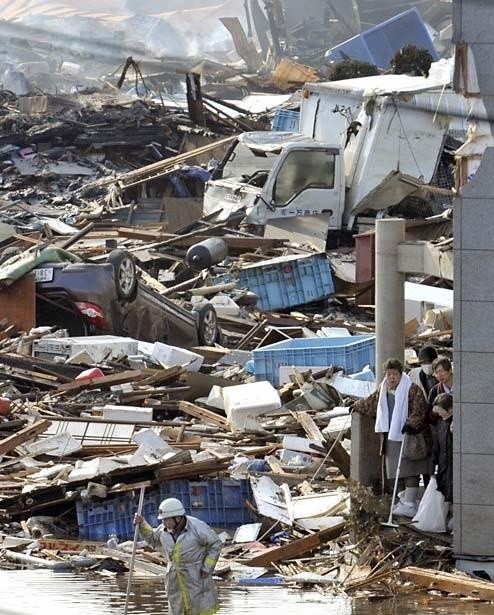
xmin=392 ymin=502 xmax=416 ymax=517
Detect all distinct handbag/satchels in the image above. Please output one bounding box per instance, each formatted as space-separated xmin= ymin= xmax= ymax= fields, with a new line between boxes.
xmin=412 ymin=476 xmax=449 ymax=532
xmin=404 ymin=434 xmax=427 ymax=460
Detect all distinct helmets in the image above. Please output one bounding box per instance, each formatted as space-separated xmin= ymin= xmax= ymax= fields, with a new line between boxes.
xmin=157 ymin=498 xmax=185 ymax=519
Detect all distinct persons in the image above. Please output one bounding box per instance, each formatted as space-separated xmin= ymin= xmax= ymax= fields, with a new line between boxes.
xmin=349 ymin=346 xmax=454 ymax=534
xmin=132 ymin=497 xmax=223 ymax=614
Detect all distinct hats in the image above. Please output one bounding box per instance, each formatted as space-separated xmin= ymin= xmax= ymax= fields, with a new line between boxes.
xmin=419 ymin=347 xmax=438 ymax=360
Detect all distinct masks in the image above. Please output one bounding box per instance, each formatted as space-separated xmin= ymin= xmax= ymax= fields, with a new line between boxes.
xmin=421 ymin=364 xmax=433 ymax=375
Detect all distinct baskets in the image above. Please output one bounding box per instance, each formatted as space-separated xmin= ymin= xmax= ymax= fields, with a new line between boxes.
xmin=272 ymin=109 xmax=300 ymax=133
